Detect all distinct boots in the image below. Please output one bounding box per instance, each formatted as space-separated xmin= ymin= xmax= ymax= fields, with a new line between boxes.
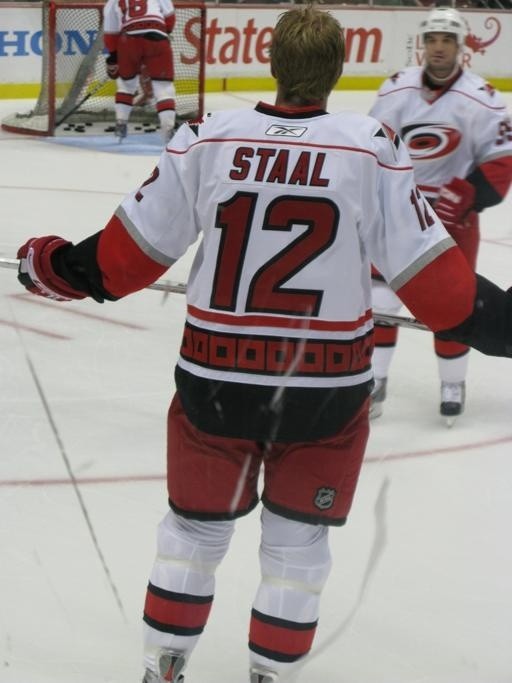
xmin=115 ymin=121 xmax=127 ymax=138
xmin=441 ymin=377 xmax=466 ymax=417
xmin=367 ymin=377 xmax=389 ymax=410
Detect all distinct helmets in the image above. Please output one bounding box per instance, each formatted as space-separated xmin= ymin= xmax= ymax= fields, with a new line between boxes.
xmin=418 ymin=6 xmax=465 ymax=47
xmin=106 ymin=57 xmax=118 ymax=80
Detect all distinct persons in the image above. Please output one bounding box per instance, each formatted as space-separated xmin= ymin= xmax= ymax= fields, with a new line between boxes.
xmin=103 ymin=0 xmax=176 ymax=140
xmin=366 ymin=8 xmax=511 ymax=427
xmin=16 ymin=8 xmax=511 ymax=683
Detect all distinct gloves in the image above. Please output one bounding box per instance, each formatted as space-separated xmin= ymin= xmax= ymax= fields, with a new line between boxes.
xmin=435 ymin=175 xmax=473 ymax=229
xmin=17 ymin=235 xmax=91 ymax=302
